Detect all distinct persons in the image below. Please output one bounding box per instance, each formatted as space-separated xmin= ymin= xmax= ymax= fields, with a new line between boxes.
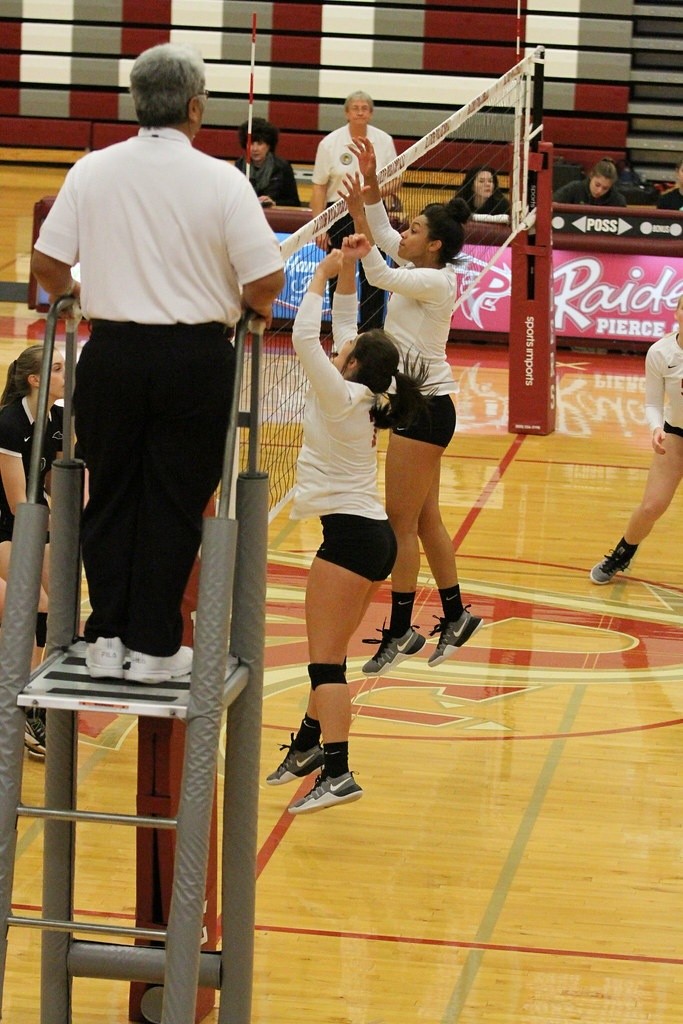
xmin=589 ymin=294 xmax=683 ymax=584
xmin=448 ymin=166 xmax=511 ymax=216
xmin=32 ymin=44 xmax=288 ymax=685
xmin=310 ymin=92 xmax=402 ymax=356
xmin=236 ymin=116 xmax=302 ymax=208
xmin=656 ymin=162 xmax=683 ymax=210
xmin=336 ymin=135 xmax=484 ymax=676
xmin=267 ymin=232 xmax=442 ymax=815
xmin=553 ymin=157 xmax=627 ymax=207
xmin=0 ymin=343 xmax=64 ymax=759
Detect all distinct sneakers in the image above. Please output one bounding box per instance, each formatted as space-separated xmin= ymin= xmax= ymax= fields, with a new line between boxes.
xmin=590 ymin=549 xmax=631 ymax=585
xmin=287 ymin=768 xmax=362 ymax=815
xmin=266 ymin=732 xmax=325 ymax=785
xmin=428 ymin=604 xmax=484 ymax=667
xmin=86 ymin=637 xmax=125 ymax=679
xmin=123 ymin=645 xmax=193 ymax=684
xmin=362 ymin=617 xmax=426 ymax=676
xmin=23 ymin=707 xmax=46 ymax=757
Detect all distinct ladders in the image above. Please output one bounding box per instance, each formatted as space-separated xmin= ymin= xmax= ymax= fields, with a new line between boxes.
xmin=1 ymin=293 xmax=268 ymax=1024
